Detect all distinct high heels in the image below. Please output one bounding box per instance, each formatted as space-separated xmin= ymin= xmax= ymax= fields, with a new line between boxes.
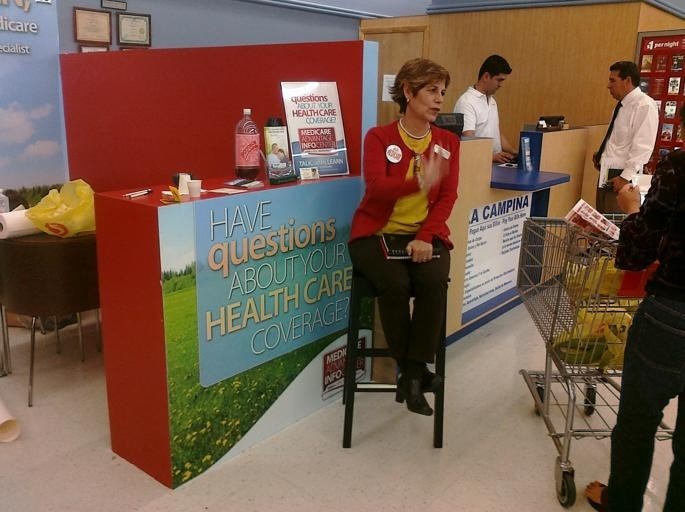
xmin=394 ymin=370 xmax=434 ymax=417
xmin=422 ymin=366 xmax=443 ymax=394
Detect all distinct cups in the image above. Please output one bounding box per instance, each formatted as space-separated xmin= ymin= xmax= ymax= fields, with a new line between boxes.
xmin=178 ymin=175 xmax=190 ymax=194
xmin=187 ymin=180 xmax=202 ymax=198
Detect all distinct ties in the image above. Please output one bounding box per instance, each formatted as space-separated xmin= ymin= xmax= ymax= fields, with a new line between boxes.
xmin=594 ymin=102 xmax=622 ymax=162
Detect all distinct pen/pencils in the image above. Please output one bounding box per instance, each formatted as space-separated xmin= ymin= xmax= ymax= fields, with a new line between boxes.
xmin=122 ymin=188 xmax=151 ymax=197
xmin=130 ymin=192 xmax=148 ymax=198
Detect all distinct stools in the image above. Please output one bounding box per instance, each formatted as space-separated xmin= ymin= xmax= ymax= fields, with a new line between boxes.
xmin=342 ymin=264 xmax=451 ymax=448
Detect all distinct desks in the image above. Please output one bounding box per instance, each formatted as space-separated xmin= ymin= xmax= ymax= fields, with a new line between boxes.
xmin=373 ymin=121 xmax=610 ymax=383
xmin=0 ymin=233 xmax=100 ymax=407
xmin=93 ymin=174 xmax=362 ymax=489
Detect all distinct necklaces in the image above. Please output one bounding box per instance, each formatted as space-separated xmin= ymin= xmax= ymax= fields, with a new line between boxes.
xmin=399 ymin=117 xmax=431 ymax=139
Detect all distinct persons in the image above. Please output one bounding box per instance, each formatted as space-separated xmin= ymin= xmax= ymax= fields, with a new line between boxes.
xmin=661 ymin=127 xmax=672 ymax=139
xmin=346 ymin=57 xmax=461 ymax=417
xmin=583 ymin=100 xmax=685 ymax=512
xmin=593 ymin=60 xmax=660 ymax=214
xmin=452 ymin=54 xmax=519 ymax=164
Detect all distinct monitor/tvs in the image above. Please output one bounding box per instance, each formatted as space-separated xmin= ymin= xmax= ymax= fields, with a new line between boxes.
xmin=432 ymin=113 xmax=464 ymax=138
xmin=536 ymin=116 xmax=565 ymax=129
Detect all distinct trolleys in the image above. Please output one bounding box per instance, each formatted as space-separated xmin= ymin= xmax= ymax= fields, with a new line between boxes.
xmin=516 ymin=213 xmax=676 ymax=508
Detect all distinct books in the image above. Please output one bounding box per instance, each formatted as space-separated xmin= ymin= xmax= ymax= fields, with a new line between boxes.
xmin=378 ymin=232 xmax=443 ymax=260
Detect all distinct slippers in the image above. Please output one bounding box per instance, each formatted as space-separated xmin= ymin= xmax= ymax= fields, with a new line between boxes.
xmin=586 ymin=483 xmax=609 ymax=512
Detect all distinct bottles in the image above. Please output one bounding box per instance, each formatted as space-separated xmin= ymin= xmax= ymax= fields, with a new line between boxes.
xmin=234 ymin=108 xmax=261 ymax=180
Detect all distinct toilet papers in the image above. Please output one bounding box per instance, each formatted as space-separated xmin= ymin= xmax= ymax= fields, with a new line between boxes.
xmin=0 ymin=209 xmax=44 ymax=241
